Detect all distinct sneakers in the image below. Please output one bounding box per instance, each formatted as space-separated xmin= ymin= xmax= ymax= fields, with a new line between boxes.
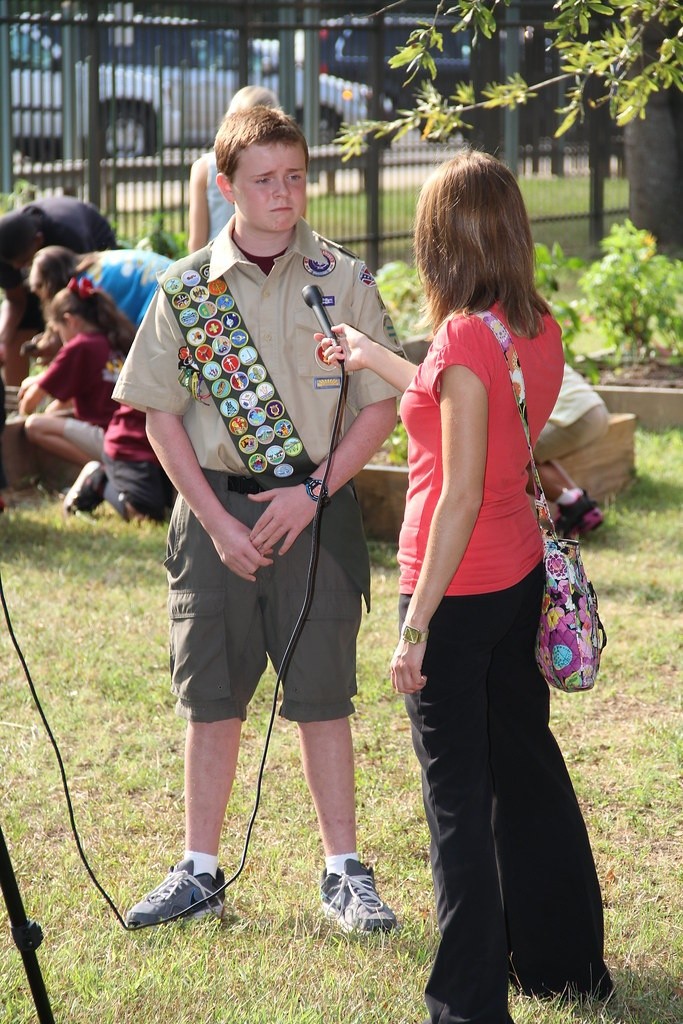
xmin=127 ymin=859 xmax=225 ymax=926
xmin=545 ymin=488 xmax=604 ymax=541
xmin=321 ymin=858 xmax=396 ymax=936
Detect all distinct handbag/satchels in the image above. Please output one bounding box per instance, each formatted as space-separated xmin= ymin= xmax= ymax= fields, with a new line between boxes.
xmin=535 ymin=538 xmax=607 ymax=692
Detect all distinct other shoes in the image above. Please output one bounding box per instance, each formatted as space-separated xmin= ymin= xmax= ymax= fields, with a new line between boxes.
xmin=63 ymin=460 xmax=104 ymax=513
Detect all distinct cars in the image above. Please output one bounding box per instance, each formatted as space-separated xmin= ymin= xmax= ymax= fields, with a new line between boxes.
xmin=8 ymin=10 xmax=394 ymax=164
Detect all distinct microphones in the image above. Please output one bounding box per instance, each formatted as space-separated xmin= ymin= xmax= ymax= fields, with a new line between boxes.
xmin=301 ymin=283 xmax=345 ymax=366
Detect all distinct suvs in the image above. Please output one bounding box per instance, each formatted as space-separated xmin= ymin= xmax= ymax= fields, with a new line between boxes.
xmin=291 ymin=13 xmax=577 ymax=152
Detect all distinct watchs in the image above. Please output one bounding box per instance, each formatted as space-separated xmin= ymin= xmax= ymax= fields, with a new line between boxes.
xmin=301 ymin=475 xmax=329 ymax=503
xmin=401 ymin=624 xmax=429 ymax=644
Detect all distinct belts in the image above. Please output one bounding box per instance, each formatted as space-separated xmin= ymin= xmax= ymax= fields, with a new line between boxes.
xmin=229 ymin=476 xmax=267 ymax=495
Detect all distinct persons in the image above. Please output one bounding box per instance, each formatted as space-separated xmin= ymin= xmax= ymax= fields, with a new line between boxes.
xmin=126 ymin=106 xmax=408 ymax=938
xmin=0 ymin=196 xmax=180 ymax=524
xmin=189 ymin=86 xmax=278 ymax=256
xmin=313 ymin=151 xmax=618 ymax=1024
xmin=526 ymin=362 xmax=609 ymax=538
